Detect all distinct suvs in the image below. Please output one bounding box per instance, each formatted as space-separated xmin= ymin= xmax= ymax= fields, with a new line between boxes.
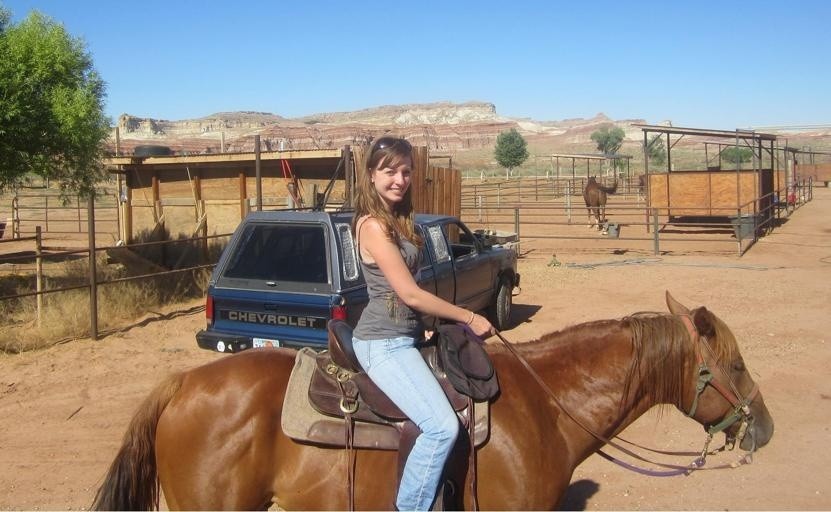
xmin=196 ymin=210 xmax=519 ymax=351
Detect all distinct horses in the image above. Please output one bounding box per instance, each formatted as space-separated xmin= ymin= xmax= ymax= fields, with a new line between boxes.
xmin=583 ymin=174 xmax=618 ymax=235
xmin=87 ymin=289 xmax=775 ymax=512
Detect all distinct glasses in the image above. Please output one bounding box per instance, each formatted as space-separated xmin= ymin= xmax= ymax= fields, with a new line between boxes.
xmin=372 ymin=137 xmax=412 ymax=153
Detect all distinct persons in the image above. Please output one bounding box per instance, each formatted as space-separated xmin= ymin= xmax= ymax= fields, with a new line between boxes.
xmin=352 ymin=137 xmax=496 ymax=512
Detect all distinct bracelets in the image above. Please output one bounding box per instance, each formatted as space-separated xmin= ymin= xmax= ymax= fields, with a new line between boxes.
xmin=467 ymin=311 xmax=474 ymax=328
